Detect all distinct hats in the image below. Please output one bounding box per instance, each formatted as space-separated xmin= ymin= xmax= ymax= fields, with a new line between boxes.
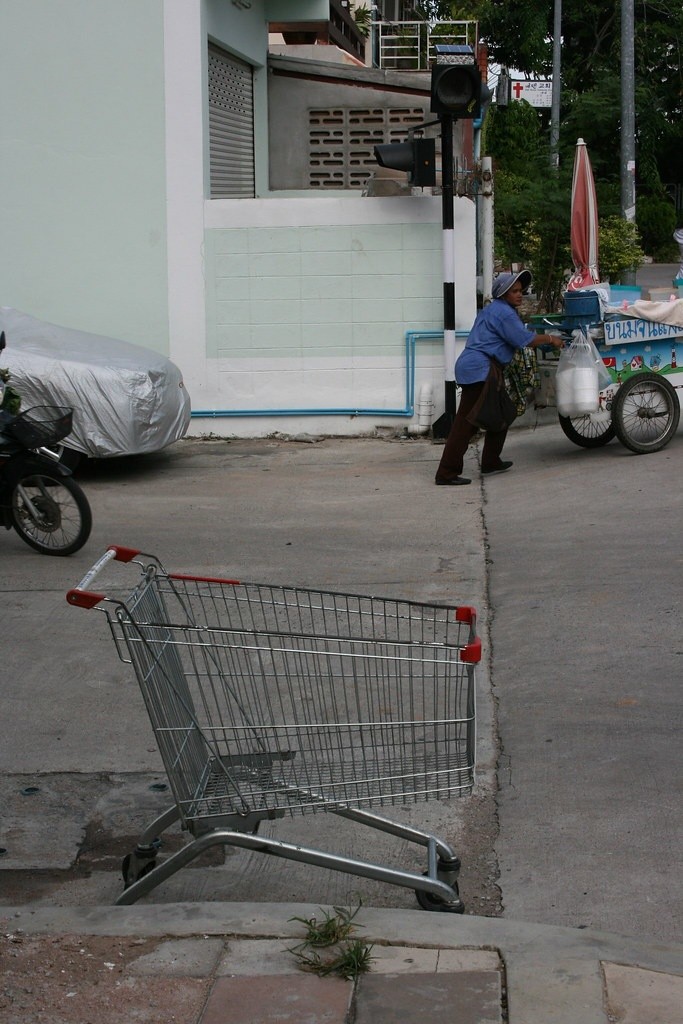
xmin=492 ymin=270 xmax=532 ymax=299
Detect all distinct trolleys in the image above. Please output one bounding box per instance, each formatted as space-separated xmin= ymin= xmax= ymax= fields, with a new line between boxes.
xmin=64 ymin=544 xmax=482 ymax=916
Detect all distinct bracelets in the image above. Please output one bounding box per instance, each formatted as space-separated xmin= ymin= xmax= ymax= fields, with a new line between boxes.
xmin=549 ymin=334 xmax=552 ymax=343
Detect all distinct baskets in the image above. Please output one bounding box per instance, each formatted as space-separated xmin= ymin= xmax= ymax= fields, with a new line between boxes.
xmin=6 ymin=405 xmax=74 ymax=446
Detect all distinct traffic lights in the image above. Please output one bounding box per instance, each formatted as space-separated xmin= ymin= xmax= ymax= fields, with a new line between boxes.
xmin=429 ymin=61 xmax=481 ymax=120
xmin=373 ymin=136 xmax=438 ymax=188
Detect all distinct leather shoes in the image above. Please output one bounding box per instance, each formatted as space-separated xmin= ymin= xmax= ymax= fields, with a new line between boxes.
xmin=499 ymin=461 xmax=513 ymax=470
xmin=440 ymin=478 xmax=472 ymax=485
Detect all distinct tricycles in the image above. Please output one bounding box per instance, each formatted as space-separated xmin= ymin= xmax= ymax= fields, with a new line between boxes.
xmin=525 ymin=280 xmax=683 ymax=454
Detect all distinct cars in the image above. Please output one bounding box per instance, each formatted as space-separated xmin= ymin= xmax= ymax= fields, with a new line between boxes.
xmin=0 ymin=304 xmax=192 ymax=485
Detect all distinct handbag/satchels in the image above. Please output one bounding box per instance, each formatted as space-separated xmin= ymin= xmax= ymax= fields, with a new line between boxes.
xmin=558 ymin=332 xmax=599 ymax=416
xmin=581 ymin=331 xmax=612 ymax=390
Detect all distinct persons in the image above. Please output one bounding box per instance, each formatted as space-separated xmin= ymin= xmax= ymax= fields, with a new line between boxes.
xmin=434 ymin=270 xmax=564 ymax=484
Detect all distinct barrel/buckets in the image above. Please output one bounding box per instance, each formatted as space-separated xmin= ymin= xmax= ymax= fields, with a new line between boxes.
xmin=561 ymin=291 xmax=600 ymax=337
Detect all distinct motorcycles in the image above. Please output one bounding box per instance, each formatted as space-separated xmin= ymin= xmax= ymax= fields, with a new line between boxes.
xmin=0 ymin=330 xmax=94 ymax=556
